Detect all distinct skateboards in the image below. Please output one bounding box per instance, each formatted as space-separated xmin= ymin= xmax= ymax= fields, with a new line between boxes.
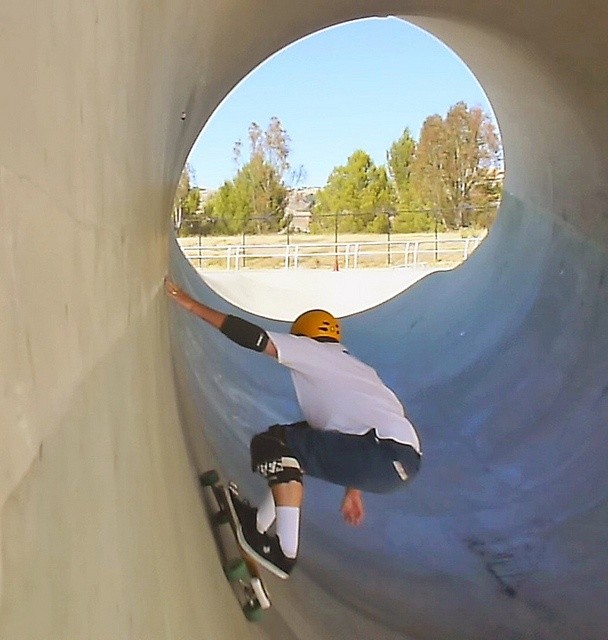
xmin=202 ymin=466 xmax=272 ymax=623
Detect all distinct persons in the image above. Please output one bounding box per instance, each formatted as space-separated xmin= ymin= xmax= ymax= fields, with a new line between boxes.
xmin=165 ymin=278 xmax=423 ymax=581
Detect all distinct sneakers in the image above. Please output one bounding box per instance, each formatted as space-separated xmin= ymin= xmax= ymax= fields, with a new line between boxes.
xmin=220 ymin=485 xmax=266 ymax=538
xmin=236 ymin=519 xmax=296 ymax=579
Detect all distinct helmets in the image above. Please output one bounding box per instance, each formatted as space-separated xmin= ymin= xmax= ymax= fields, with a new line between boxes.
xmin=290 ymin=310 xmax=340 ymax=342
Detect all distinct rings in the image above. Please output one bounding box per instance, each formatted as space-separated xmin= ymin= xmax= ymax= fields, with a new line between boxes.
xmin=172 ymin=291 xmax=177 ymax=295
